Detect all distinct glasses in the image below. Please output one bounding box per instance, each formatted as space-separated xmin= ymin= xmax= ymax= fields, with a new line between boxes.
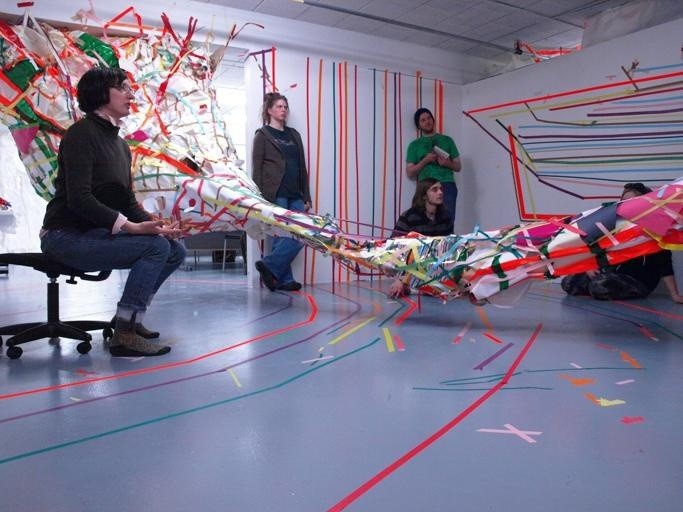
xmin=108 ymin=83 xmax=137 ymax=95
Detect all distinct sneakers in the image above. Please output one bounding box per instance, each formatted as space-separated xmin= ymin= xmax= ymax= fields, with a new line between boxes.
xmin=108 ymin=318 xmax=172 ymax=357
xmin=110 ymin=315 xmax=160 ymax=340
xmin=277 ymin=280 xmax=302 ymax=291
xmin=255 ymin=259 xmax=277 ymax=292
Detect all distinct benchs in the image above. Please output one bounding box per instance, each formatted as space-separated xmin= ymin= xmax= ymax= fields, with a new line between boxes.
xmin=178 ymin=228 xmax=247 ymax=275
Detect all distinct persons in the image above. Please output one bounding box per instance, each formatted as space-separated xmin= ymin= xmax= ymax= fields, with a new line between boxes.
xmin=34 ymin=64 xmax=186 ymax=356
xmin=402 ymin=107 xmax=462 ymax=223
xmin=384 ymin=178 xmax=454 ymax=299
xmin=561 ymin=180 xmax=682 ymax=307
xmin=252 ymin=91 xmax=312 ymax=293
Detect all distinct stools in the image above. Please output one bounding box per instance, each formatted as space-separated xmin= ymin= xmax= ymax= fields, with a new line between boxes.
xmin=0 ymin=251 xmax=117 ymax=361
